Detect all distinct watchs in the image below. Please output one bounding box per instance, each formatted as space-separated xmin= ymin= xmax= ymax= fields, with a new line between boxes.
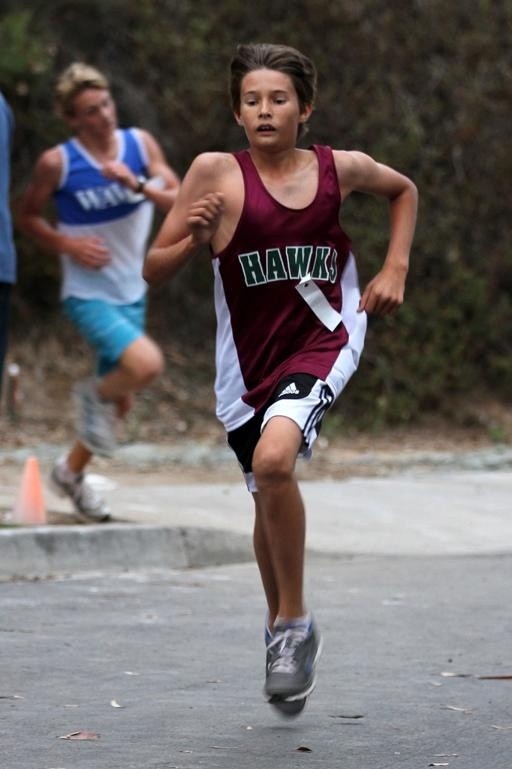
xmin=134 ymin=175 xmax=147 ymax=194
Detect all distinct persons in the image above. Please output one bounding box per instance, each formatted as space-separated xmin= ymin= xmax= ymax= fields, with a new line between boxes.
xmin=14 ymin=60 xmax=182 ymax=528
xmin=141 ymin=40 xmax=421 ymax=703
xmin=0 ymin=94 xmax=19 ymax=373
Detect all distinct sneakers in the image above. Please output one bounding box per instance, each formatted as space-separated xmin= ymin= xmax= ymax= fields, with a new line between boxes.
xmin=263 ymin=614 xmax=323 ymax=721
xmin=71 ymin=377 xmax=118 ymax=457
xmin=48 ymin=454 xmax=111 ymax=525
